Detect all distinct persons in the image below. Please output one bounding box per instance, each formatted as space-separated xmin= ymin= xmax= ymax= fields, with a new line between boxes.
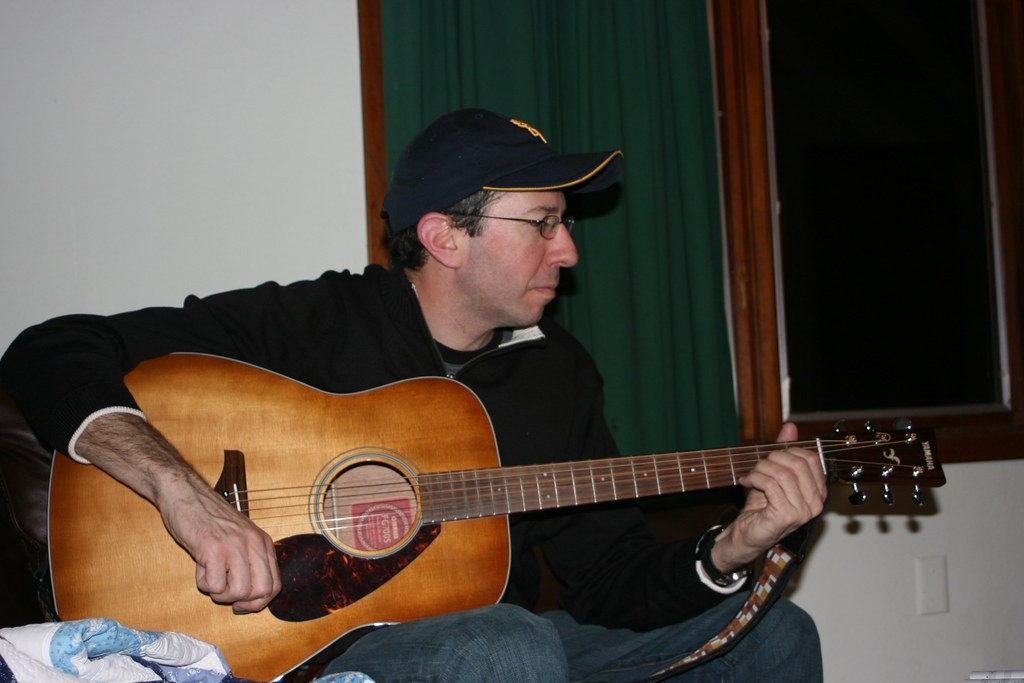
xmin=0 ymin=108 xmax=830 ymax=683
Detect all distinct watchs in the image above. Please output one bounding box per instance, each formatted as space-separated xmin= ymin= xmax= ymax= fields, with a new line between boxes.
xmin=695 ymin=527 xmax=750 ymax=588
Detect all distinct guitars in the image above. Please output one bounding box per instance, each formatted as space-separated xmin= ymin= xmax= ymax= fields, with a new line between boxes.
xmin=44 ymin=348 xmax=949 ymax=683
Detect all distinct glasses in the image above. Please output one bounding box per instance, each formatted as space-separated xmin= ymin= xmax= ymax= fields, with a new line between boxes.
xmin=447 ymin=211 xmax=575 ymax=240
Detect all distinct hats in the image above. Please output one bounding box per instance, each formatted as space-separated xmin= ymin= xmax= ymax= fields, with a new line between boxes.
xmin=379 ymin=107 xmax=625 ymax=238
